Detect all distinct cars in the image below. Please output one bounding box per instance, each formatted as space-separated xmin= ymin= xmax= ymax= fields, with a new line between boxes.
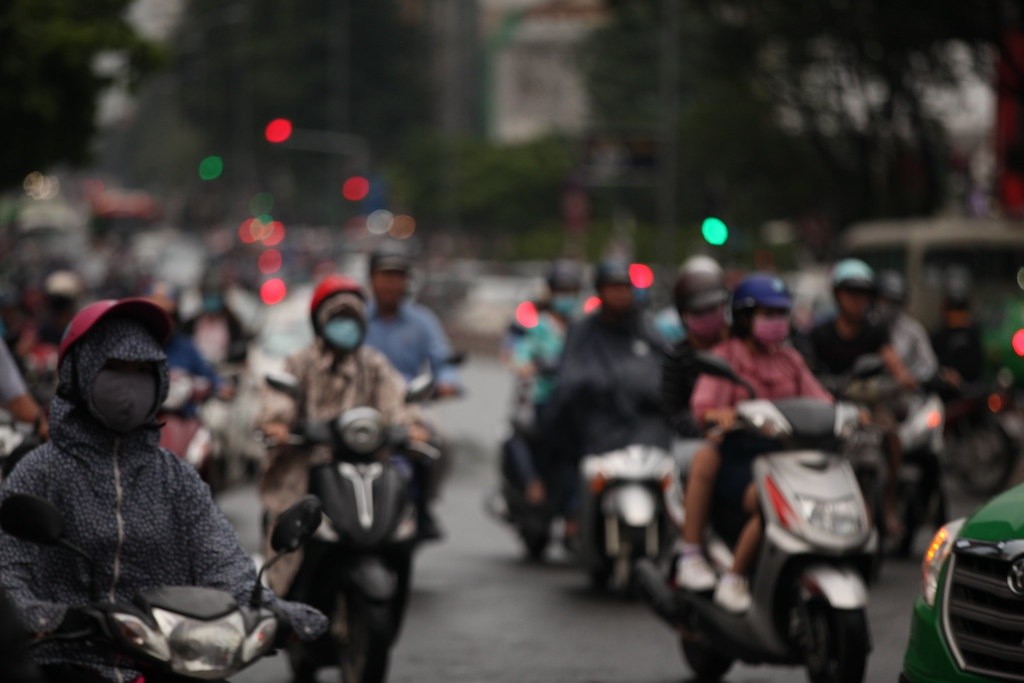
xmin=898 ymin=479 xmax=1024 ymax=683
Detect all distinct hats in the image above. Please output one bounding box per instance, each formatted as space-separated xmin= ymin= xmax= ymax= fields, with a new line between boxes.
xmin=59 ymin=297 xmax=174 ymax=378
xmin=312 ymin=275 xmax=367 ymax=318
xmin=371 ymin=240 xmax=412 ymax=274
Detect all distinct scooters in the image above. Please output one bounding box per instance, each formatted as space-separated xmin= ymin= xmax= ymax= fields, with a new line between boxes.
xmin=805 ymin=351 xmax=914 ymax=589
xmin=632 ymin=345 xmax=888 ymax=683
xmin=880 ymin=364 xmax=960 ymax=556
xmin=0 ymin=336 xmax=253 ymax=513
xmin=493 ymin=346 xmax=675 ymax=602
xmin=0 ymin=494 xmax=325 ymax=683
xmin=253 ymin=347 xmax=472 ymax=683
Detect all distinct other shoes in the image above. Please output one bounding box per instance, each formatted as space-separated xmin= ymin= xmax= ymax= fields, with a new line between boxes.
xmin=676 ymin=553 xmax=718 ymax=590
xmin=713 ymin=571 xmax=753 ymax=613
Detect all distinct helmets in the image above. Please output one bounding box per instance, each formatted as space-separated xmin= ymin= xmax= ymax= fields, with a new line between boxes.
xmin=593 ymin=257 xmax=636 ymax=286
xmin=732 ymin=272 xmax=794 ymax=310
xmin=674 ymin=255 xmax=731 ymax=314
xmin=942 ymin=264 xmax=975 ymax=307
xmin=832 ymin=258 xmax=879 ymax=293
xmin=879 ymin=269 xmax=908 ymax=304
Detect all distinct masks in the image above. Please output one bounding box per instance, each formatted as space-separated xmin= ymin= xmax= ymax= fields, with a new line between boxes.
xmin=92 ymin=368 xmax=156 ymax=432
xmin=549 ymin=291 xmax=578 ymax=313
xmin=687 ymin=309 xmax=723 ymax=339
xmin=322 ymin=315 xmax=363 ymax=350
xmin=752 ymin=313 xmax=790 ymax=346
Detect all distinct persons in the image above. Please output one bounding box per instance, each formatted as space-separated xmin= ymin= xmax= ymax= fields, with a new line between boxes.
xmin=499 ymin=252 xmax=1024 ymax=617
xmin=0 ymin=187 xmax=462 ymax=683
